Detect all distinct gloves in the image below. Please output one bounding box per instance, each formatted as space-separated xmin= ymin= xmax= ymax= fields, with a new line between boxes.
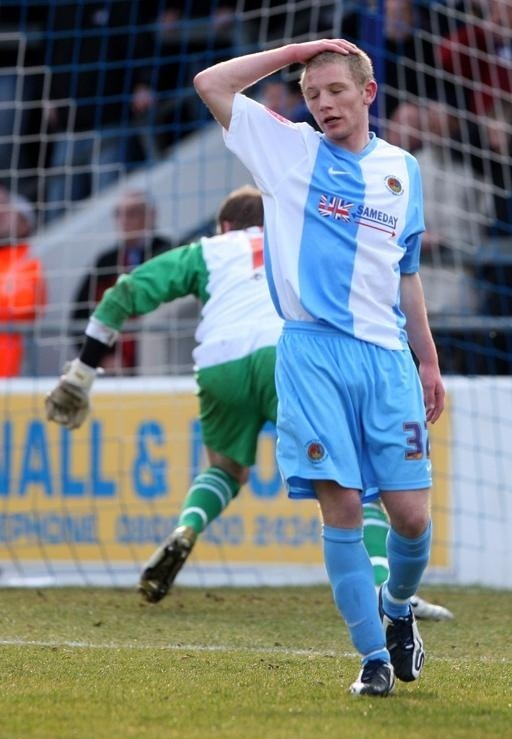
xmin=45 ymin=357 xmax=99 ymax=430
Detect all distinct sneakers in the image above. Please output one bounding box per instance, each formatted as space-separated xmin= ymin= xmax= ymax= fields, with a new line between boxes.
xmin=349 ymin=660 xmax=396 ymax=696
xmin=377 ymin=583 xmax=425 ymax=683
xmin=137 ymin=525 xmax=195 ymax=604
xmin=405 ymin=595 xmax=454 ymax=623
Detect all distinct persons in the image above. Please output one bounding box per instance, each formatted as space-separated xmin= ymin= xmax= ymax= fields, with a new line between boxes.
xmin=0 ymin=0 xmax=511 ymax=377
xmin=42 ymin=186 xmax=456 ymax=624
xmin=195 ymin=36 xmax=447 ymax=698
